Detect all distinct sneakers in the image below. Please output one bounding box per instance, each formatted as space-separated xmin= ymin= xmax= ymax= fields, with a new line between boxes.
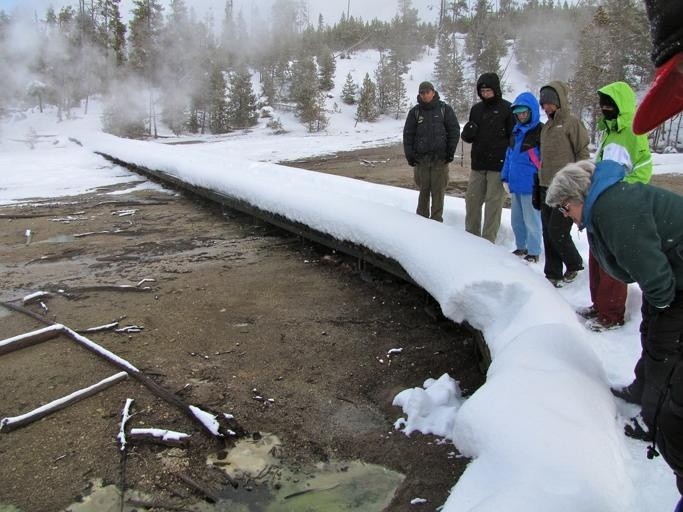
xmin=625 ymin=411 xmax=655 ymax=443
xmin=513 ymin=249 xmax=623 ymax=332
xmin=612 ymin=380 xmax=641 ymax=403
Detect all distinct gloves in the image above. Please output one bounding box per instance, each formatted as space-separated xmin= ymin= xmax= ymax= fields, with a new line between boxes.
xmin=445 ymin=153 xmax=454 ymax=164
xmin=503 ymin=182 xmax=510 ymax=194
xmin=408 ymin=159 xmax=420 ymax=166
xmin=463 ymin=122 xmax=478 ymax=139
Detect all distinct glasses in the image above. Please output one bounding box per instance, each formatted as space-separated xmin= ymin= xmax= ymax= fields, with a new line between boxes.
xmin=558 ymin=202 xmax=572 ymax=213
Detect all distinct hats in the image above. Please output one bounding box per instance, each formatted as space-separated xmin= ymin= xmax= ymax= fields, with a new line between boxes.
xmin=632 ymin=0 xmax=683 ymax=134
xmin=419 ymin=81 xmax=434 ymax=93
xmin=540 ymin=88 xmax=559 ymax=106
xmin=512 ymin=106 xmax=528 ymax=114
xmin=600 ymin=95 xmax=615 ymax=107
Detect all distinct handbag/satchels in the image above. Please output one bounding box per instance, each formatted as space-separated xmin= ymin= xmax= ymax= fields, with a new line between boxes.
xmin=532 ymin=174 xmax=540 ymax=210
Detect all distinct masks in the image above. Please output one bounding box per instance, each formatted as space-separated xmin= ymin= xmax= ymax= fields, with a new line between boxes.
xmin=602 ymin=109 xmax=614 ymax=119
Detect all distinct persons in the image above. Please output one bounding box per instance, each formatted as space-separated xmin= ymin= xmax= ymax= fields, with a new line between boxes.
xmin=640 ymin=1 xmax=683 ymax=511
xmin=574 ymin=81 xmax=652 ymax=332
xmin=545 ymin=157 xmax=682 ymax=441
xmin=460 ymin=72 xmax=517 ymax=241
xmin=500 ymin=91 xmax=543 ymax=264
xmin=402 ymin=82 xmax=460 ymax=223
xmin=539 ymin=79 xmax=591 ymax=288
